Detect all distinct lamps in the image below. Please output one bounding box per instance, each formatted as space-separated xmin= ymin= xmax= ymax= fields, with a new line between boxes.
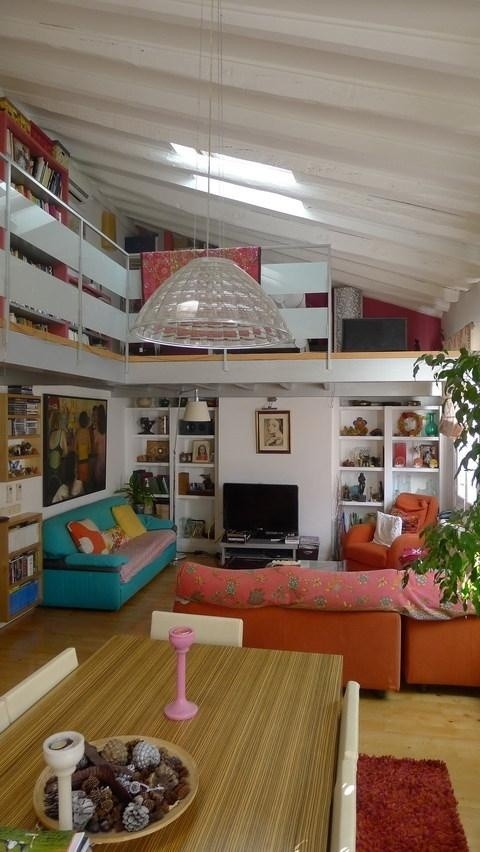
xmin=131 ymin=0 xmax=295 ymax=348
xmin=172 ymin=389 xmax=210 ymax=532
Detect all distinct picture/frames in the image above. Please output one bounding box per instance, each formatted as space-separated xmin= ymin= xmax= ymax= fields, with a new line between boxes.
xmin=43 ymin=394 xmax=107 ymax=506
xmin=192 ymin=440 xmax=210 ymax=463
xmin=257 ymin=411 xmax=290 ymax=454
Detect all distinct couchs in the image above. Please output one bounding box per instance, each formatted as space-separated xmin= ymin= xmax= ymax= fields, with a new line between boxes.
xmin=41 ymin=497 xmax=178 ymax=608
xmin=173 ymin=557 xmax=479 ymax=689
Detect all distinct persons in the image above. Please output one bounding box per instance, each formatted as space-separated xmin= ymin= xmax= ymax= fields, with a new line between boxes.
xmin=196 ymin=445 xmax=208 ymax=459
xmin=265 ymin=419 xmax=283 ymax=447
xmin=45 ymin=403 xmax=105 ymax=505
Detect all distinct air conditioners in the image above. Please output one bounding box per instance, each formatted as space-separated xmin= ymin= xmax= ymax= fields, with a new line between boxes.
xmin=69 ymin=173 xmax=91 ymax=205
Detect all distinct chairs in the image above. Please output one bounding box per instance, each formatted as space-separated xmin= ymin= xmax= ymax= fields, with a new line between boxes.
xmin=342 ymin=493 xmax=437 ymax=571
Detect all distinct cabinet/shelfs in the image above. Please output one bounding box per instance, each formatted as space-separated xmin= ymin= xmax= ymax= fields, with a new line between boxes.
xmin=125 ymin=407 xmax=215 ymax=552
xmin=220 ymin=537 xmax=296 ymax=567
xmin=340 ymin=408 xmax=439 ymax=531
xmin=0 ymin=112 xmax=68 ymax=343
xmin=0 ymin=512 xmax=44 ymax=623
xmin=0 ymin=393 xmax=43 ymax=482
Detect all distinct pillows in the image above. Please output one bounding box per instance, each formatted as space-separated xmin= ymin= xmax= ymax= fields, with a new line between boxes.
xmin=372 ymin=511 xmax=402 ymax=547
xmin=68 ymin=506 xmax=146 ymax=555
xmin=391 ymin=509 xmax=419 ymax=533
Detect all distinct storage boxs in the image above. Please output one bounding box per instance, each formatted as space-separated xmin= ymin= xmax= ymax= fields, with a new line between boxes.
xmin=0 ymin=96 xmax=71 ymax=172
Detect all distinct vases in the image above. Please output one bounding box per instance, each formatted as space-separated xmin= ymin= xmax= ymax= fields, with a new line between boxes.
xmin=425 ymin=412 xmax=438 ymax=437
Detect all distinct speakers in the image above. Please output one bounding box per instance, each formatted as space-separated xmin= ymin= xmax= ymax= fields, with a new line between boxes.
xmin=341 ymin=316 xmax=409 ymax=352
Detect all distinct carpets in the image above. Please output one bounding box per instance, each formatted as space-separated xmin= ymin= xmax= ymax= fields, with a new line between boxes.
xmin=358 ymin=755 xmax=470 ymax=852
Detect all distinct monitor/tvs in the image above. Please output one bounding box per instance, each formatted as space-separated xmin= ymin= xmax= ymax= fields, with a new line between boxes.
xmin=221 ymin=482 xmax=299 ymax=540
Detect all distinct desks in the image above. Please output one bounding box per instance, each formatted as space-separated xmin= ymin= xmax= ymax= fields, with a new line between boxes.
xmin=0 ymin=610 xmax=359 ymax=852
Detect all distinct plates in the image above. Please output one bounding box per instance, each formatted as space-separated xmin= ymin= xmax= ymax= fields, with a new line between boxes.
xmin=34 ymin=735 xmax=199 ymax=844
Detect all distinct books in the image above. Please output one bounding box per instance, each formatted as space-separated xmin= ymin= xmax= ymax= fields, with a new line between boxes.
xmin=8 ymin=383 xmax=40 ymax=435
xmin=9 ymin=522 xmax=40 ymax=612
xmin=3 ymin=128 xmax=65 ymax=331
